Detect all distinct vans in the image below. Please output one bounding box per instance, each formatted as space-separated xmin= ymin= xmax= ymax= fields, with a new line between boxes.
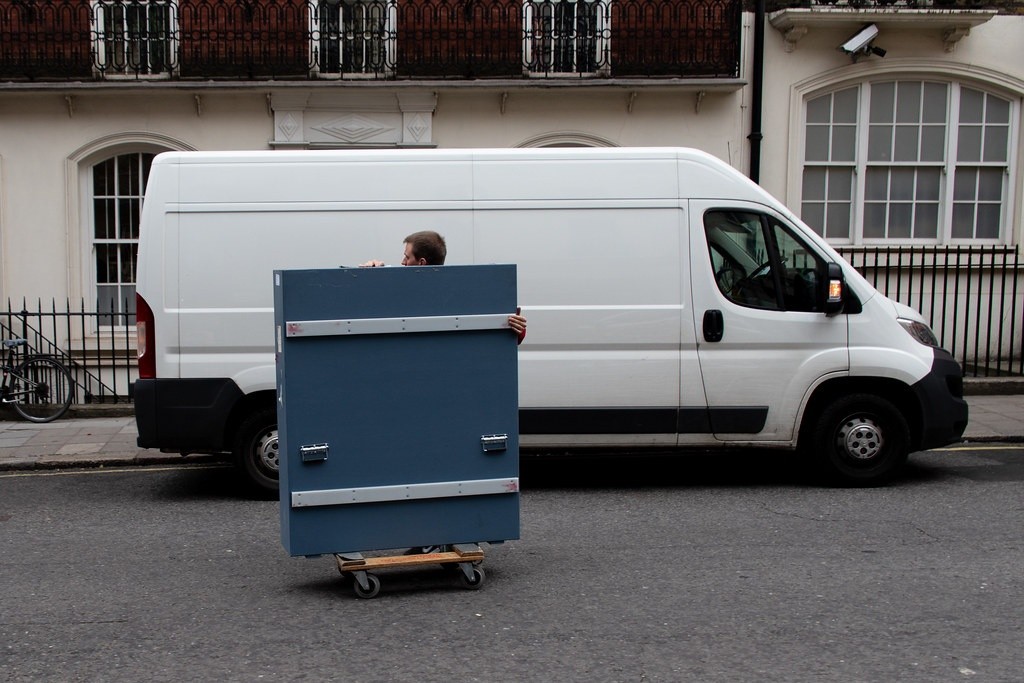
xmin=132 ymin=145 xmax=973 ymax=505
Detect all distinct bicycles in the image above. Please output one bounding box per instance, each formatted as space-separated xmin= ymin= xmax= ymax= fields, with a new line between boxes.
xmin=0 ymin=338 xmax=77 ymax=424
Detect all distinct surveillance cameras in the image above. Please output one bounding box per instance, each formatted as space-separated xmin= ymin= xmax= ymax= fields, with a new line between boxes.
xmin=836 ymin=22 xmax=879 ymax=55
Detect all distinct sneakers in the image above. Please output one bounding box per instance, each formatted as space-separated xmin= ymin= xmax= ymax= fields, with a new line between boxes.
xmin=403 ymin=546 xmax=441 ymax=556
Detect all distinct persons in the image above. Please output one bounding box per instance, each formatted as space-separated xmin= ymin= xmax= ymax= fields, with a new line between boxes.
xmin=358 ymin=230 xmax=528 ymax=346
